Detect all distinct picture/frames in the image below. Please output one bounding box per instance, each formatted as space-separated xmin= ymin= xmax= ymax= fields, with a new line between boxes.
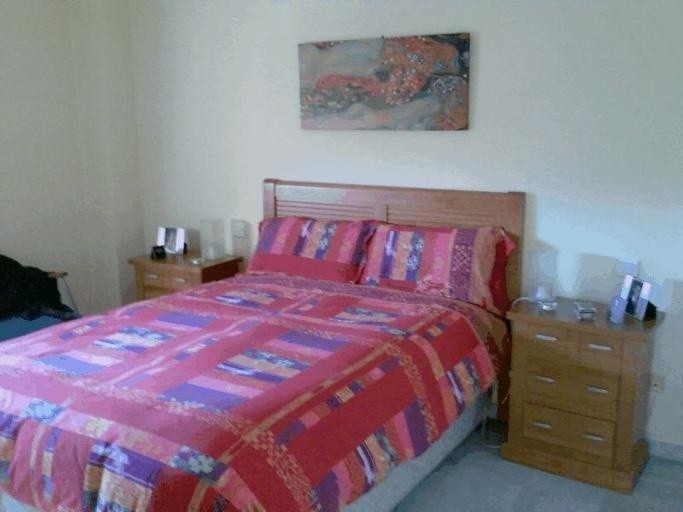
xmin=155 ymin=225 xmax=187 ymax=256
xmin=616 ymin=272 xmax=656 ymax=322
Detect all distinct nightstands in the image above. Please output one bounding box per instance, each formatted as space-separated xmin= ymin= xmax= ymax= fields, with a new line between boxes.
xmin=499 ymin=292 xmax=666 ymax=496
xmin=125 ymin=250 xmax=245 ymax=304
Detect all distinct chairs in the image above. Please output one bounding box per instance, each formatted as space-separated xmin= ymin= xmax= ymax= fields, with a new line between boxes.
xmin=0 ymin=253 xmax=82 ymax=343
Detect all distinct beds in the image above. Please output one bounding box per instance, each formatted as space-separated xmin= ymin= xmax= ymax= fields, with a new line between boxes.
xmin=0 ymin=177 xmax=526 ymax=511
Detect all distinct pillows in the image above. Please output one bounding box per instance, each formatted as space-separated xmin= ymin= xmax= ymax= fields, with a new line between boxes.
xmin=352 ymin=218 xmax=516 ymax=319
xmin=246 ymin=213 xmax=378 ymax=284
